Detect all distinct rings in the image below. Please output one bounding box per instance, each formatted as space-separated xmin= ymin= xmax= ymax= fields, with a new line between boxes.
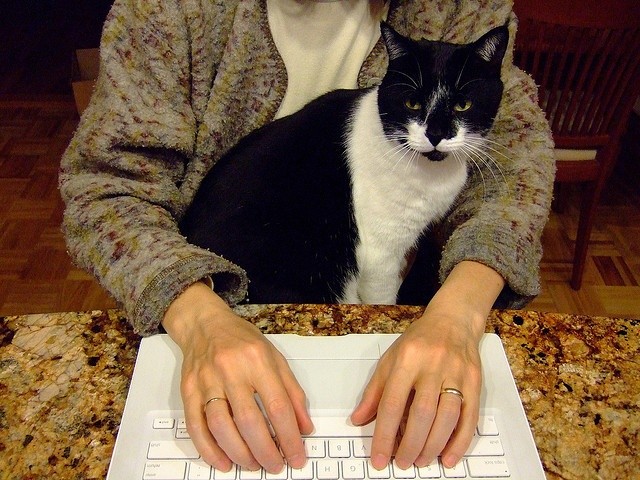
xmin=440 ymin=388 xmax=464 ymax=401
xmin=203 ymin=396 xmax=229 ymax=413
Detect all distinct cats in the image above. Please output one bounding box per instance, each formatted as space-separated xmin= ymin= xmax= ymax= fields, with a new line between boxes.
xmin=178 ymin=18 xmax=509 ymax=305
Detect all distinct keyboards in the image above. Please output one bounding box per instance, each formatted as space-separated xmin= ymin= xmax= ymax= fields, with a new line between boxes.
xmin=107 ymin=333 xmax=548 ymax=480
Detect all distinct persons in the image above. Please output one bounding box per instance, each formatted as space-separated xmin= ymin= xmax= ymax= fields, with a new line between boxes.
xmin=57 ymin=0 xmax=557 ymax=474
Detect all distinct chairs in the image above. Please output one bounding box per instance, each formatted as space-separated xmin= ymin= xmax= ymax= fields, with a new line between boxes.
xmin=505 ymin=0 xmax=640 ymax=292
